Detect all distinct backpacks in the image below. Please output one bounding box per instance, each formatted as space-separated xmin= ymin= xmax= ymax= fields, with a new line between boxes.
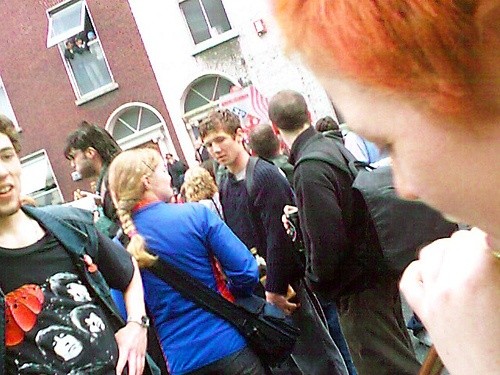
xmin=291 ymin=139 xmax=459 ymax=272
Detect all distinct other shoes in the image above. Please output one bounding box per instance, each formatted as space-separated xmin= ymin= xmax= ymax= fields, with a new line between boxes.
xmin=419 ymin=332 xmax=433 ymax=348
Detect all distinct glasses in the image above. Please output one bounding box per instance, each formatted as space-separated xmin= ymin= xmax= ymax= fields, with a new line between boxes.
xmin=65 ymin=149 xmax=82 ymax=161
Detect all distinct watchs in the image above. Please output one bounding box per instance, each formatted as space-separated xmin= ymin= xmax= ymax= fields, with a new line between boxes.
xmin=126 ymin=314 xmax=151 ymax=329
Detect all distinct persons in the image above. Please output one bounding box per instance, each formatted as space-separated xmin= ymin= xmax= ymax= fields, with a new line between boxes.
xmin=0 ymin=0 xmax=422 ymax=375
xmin=266 ymin=0 xmax=500 ymax=375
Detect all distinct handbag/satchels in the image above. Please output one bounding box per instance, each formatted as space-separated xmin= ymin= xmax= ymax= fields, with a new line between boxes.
xmin=238 ymin=292 xmax=299 ymax=366
